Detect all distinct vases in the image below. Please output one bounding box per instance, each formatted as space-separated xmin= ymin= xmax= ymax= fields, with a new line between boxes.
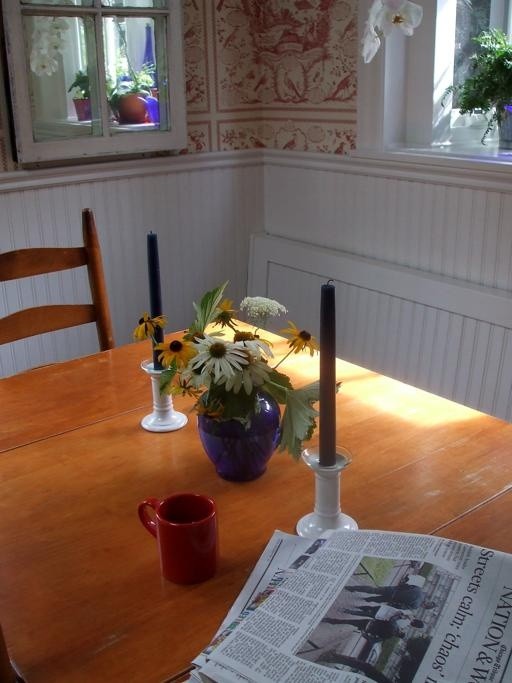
xmin=198 ymin=383 xmax=282 ymax=484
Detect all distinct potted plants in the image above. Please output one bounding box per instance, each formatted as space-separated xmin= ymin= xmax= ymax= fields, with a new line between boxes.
xmin=104 ymin=63 xmax=156 ymax=124
xmin=67 ymin=70 xmax=91 ymax=122
xmin=440 ymin=28 xmax=512 ymax=149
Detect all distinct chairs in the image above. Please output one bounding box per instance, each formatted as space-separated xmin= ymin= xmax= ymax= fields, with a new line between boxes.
xmin=0 ymin=209 xmax=116 ymax=352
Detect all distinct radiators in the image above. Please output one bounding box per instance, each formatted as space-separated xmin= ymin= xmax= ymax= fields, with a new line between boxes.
xmin=249 ymin=233 xmax=512 ymax=424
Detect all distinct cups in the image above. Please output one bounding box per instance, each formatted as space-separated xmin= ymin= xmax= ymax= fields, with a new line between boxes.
xmin=138 ymin=493 xmax=220 ymax=586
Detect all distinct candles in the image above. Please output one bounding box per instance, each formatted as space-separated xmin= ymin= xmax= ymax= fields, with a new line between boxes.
xmin=319 ymin=281 xmax=336 ymax=467
xmin=148 ymin=231 xmax=163 ymax=371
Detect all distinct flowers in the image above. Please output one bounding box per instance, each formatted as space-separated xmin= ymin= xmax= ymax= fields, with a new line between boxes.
xmin=135 ymin=280 xmax=340 ymax=461
